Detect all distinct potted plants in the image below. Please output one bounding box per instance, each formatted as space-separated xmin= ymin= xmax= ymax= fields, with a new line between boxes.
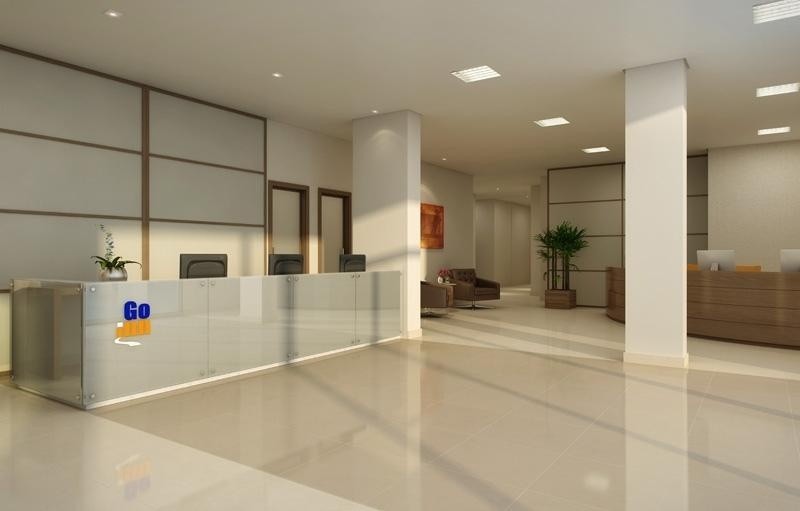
xmin=532 ymin=220 xmax=590 ymax=309
xmin=89 ymin=223 xmax=143 ymax=281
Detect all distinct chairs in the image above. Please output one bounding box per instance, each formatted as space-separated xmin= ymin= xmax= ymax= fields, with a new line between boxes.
xmin=450 ymin=268 xmax=500 ymax=310
xmin=422 ymin=281 xmax=454 ymax=318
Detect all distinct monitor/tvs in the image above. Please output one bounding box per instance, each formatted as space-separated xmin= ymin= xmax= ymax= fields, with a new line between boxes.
xmin=180 ymin=254 xmax=227 ymax=278
xmin=268 ymin=254 xmax=303 ymax=275
xmin=340 ymin=254 xmax=365 ymax=272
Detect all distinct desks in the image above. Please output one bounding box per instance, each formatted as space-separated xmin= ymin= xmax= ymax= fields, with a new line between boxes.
xmin=437 ymin=282 xmax=457 ymax=306
